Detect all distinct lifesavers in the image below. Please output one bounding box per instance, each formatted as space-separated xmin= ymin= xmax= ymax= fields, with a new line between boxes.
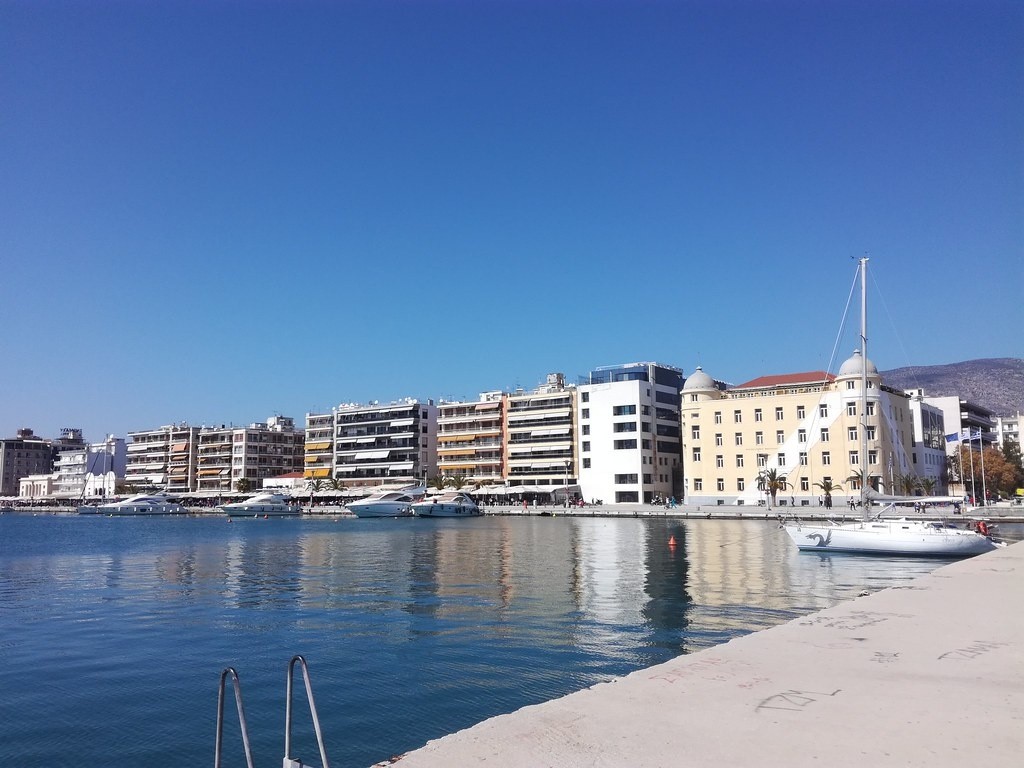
xmin=979 ymin=521 xmax=989 ymax=535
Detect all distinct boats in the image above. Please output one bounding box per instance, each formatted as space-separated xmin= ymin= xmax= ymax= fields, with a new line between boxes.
xmin=220 ymin=493 xmax=303 ymax=516
xmin=345 ymin=491 xmax=415 ymax=518
xmin=410 ymin=491 xmax=485 ymax=517
xmin=97 ymin=493 xmax=188 ymax=515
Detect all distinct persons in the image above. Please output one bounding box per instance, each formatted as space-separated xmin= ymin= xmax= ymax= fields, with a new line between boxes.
xmin=914 ymin=501 xmax=926 ymax=514
xmin=954 ymin=501 xmax=961 ymax=514
xmin=986 ymin=489 xmax=991 ymax=506
xmin=850 ymin=496 xmax=856 ymax=510
xmin=826 ymin=495 xmax=831 ymax=509
xmin=666 ymin=496 xmax=677 ymax=509
xmin=819 ymin=495 xmax=823 ymax=507
xmin=791 ymin=497 xmax=795 ymax=506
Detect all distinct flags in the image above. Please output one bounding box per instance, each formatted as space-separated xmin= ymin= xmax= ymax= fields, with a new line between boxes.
xmin=945 ymin=432 xmax=958 ymax=442
xmin=959 ymin=430 xmax=969 ymax=441
xmin=970 ymin=430 xmax=980 ymax=440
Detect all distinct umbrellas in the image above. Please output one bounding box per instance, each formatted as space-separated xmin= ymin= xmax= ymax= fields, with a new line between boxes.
xmin=288 ymin=489 xmax=372 ymax=500
xmin=178 ymin=490 xmax=259 ymax=498
xmin=424 ymin=487 xmax=558 ymax=495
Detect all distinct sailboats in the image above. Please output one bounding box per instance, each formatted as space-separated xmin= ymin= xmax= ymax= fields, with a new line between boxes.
xmin=779 ymin=258 xmax=1008 ymax=556
xmin=75 ymin=434 xmax=109 ymax=514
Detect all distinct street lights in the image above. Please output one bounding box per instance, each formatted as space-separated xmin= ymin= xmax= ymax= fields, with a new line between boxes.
xmin=219 ymin=475 xmax=222 ymax=506
xmin=422 ymin=465 xmax=430 ymax=498
xmin=309 ymin=471 xmax=314 ymax=506
